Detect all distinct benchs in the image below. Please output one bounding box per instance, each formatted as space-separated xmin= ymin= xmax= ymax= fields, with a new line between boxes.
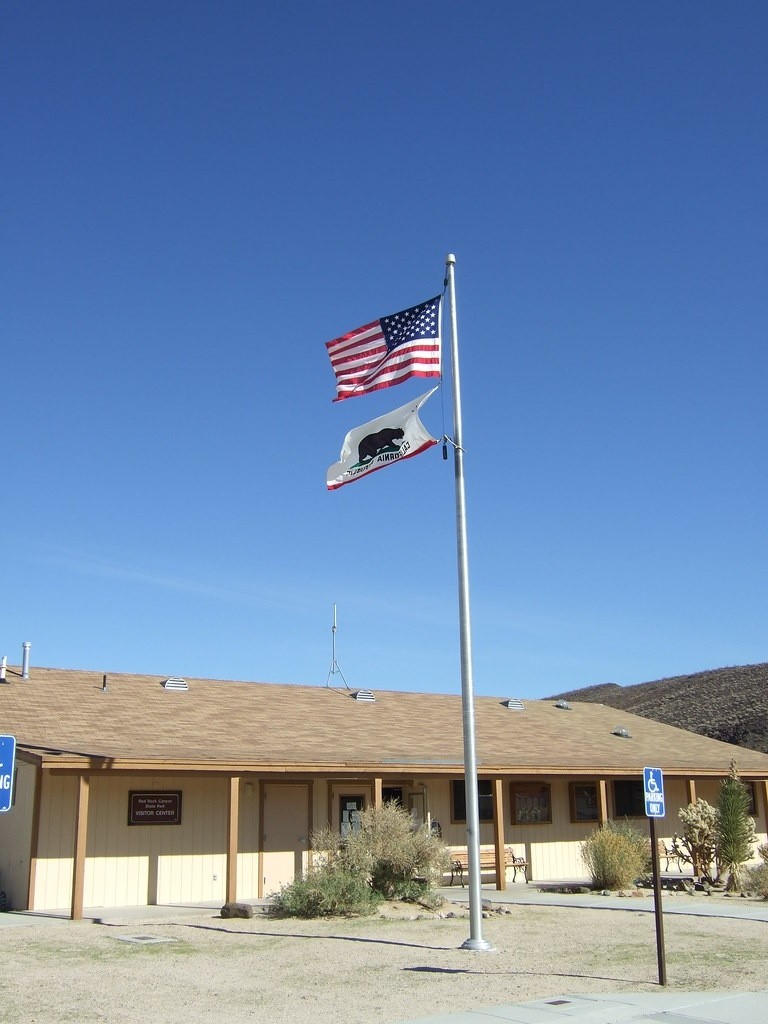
xmin=447 ymin=847 xmax=530 ymax=888
xmin=646 ymin=840 xmax=683 ymax=873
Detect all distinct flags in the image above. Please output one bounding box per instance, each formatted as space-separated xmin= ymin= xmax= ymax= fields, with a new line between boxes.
xmin=327 ymin=385 xmax=439 ymax=490
xmin=324 ymin=295 xmax=443 ymax=402
